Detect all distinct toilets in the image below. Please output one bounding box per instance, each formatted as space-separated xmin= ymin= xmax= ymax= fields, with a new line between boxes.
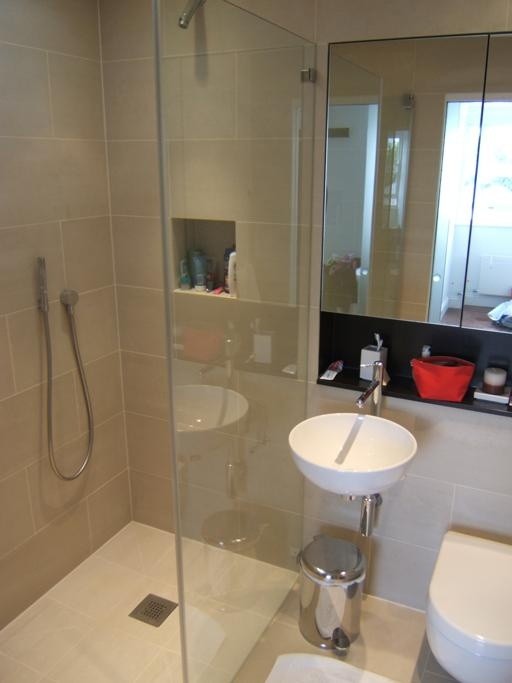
xmin=427 ymin=529 xmax=512 ymax=683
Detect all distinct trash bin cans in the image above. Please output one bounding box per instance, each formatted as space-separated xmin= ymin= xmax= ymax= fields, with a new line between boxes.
xmin=295 ymin=535 xmax=369 ymax=654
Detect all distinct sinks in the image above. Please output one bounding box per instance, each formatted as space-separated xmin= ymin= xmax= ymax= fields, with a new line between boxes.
xmin=287 ymin=412 xmax=419 ymax=495
xmin=173 ymin=383 xmax=249 ymax=458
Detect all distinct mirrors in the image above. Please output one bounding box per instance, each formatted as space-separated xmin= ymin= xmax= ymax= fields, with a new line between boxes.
xmin=319 ymin=29 xmax=512 ymax=335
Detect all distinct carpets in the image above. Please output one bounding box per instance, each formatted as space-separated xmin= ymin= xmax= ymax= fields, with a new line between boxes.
xmin=263 ymin=653 xmax=395 ymax=683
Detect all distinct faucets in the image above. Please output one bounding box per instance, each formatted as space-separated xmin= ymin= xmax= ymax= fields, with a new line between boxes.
xmin=198 ymin=357 xmax=234 ymax=389
xmin=358 ymin=361 xmax=385 ymax=418
